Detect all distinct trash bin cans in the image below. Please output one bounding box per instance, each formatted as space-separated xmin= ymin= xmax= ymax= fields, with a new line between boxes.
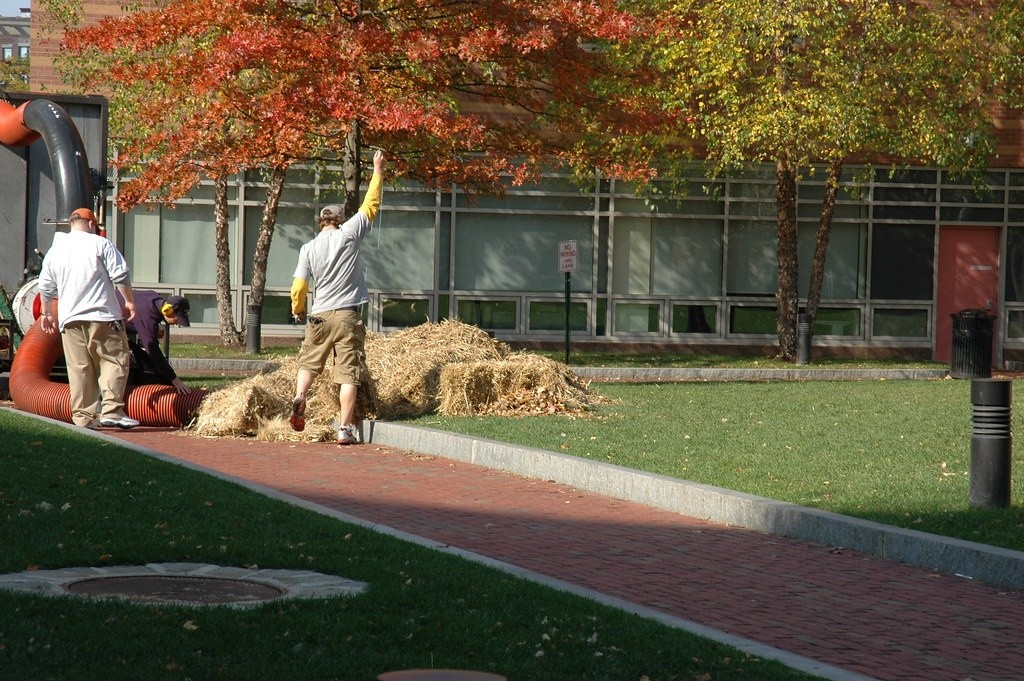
xmin=948 ymin=307 xmax=998 ymax=381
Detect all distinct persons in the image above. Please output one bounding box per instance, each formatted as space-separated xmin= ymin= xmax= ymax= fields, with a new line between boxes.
xmin=114 ymin=286 xmax=191 ymax=395
xmin=288 ymin=148 xmax=386 ymax=443
xmin=37 ymin=207 xmax=142 ymax=428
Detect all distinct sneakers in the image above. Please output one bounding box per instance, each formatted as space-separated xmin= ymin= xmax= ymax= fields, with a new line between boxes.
xmin=99 ymin=408 xmax=140 ymax=428
xmin=338 ymin=426 xmax=357 ymax=444
xmin=290 ymin=396 xmax=306 ymax=431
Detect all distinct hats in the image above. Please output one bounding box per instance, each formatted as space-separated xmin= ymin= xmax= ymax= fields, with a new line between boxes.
xmin=68 ymin=208 xmax=101 ymax=233
xmin=167 ymin=296 xmax=191 ymax=327
xmin=320 ymin=205 xmax=347 ymax=222
